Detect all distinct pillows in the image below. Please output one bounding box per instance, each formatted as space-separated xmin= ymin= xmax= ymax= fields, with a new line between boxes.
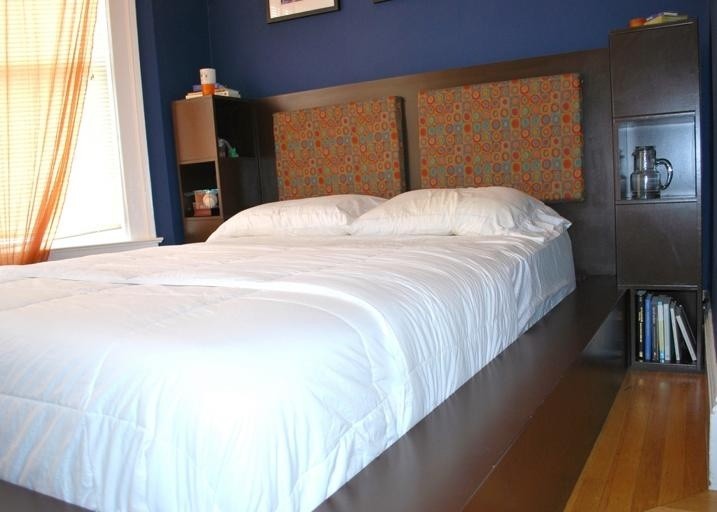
xmin=348 ymin=185 xmax=573 ymax=244
xmin=206 ymin=194 xmax=383 ymax=244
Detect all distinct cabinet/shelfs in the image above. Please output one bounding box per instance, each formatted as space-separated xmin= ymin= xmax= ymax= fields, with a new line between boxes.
xmin=172 ymin=95 xmax=253 ymax=244
xmin=607 ymin=16 xmax=706 ymax=372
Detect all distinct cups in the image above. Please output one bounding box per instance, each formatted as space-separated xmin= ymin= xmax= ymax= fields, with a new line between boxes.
xmin=200 ymin=68 xmax=217 ymax=96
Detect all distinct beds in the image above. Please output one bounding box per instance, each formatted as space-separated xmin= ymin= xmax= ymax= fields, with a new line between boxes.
xmin=0 ymin=48 xmax=614 ymax=512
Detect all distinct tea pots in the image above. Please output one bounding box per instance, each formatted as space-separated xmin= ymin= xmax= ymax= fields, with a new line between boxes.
xmin=630 ymin=145 xmax=674 ymax=199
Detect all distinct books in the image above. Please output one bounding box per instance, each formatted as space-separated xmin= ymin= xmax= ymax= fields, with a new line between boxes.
xmin=645 ymin=10 xmax=688 ymax=24
xmin=635 ymin=288 xmax=697 ymax=363
xmin=186 ymin=84 xmax=239 ymax=98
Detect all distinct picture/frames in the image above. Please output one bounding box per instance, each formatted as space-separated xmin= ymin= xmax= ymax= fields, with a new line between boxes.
xmin=265 ymin=0 xmax=340 ymax=24
